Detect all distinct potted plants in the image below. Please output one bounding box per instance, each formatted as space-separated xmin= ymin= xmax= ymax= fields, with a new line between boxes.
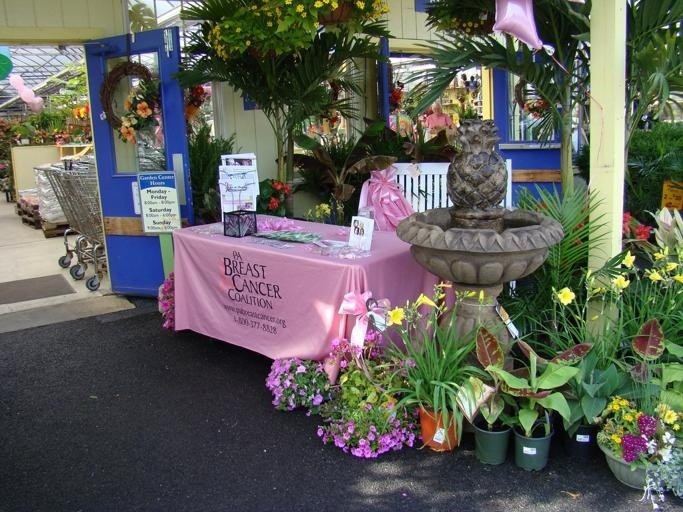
xmin=405 ymin=286 xmax=483 ymax=454
xmin=497 ymin=352 xmax=577 ymax=474
xmin=463 ymin=334 xmax=517 ymax=466
xmin=610 ymin=207 xmax=683 ymax=391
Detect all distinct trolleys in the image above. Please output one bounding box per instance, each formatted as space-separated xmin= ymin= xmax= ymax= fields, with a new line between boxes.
xmin=33 ymin=141 xmax=166 ymax=291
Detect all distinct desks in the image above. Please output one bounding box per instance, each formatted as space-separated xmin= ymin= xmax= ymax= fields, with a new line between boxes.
xmin=169 ymin=209 xmax=443 ymax=374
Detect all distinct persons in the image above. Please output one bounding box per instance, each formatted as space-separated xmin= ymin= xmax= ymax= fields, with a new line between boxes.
xmin=423 ymin=101 xmax=454 ymax=131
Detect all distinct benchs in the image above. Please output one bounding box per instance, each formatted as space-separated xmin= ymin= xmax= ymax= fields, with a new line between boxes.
xmin=366 ymin=160 xmax=517 ymax=242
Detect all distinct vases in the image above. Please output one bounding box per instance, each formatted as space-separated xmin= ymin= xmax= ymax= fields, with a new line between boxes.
xmin=598 ymin=441 xmax=675 ymax=492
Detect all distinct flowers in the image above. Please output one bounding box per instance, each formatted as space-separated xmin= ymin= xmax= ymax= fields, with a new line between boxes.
xmin=322 ymin=341 xmax=415 ymax=462
xmin=269 ymin=357 xmax=334 ymax=413
xmin=598 ymin=394 xmax=683 ymax=466
